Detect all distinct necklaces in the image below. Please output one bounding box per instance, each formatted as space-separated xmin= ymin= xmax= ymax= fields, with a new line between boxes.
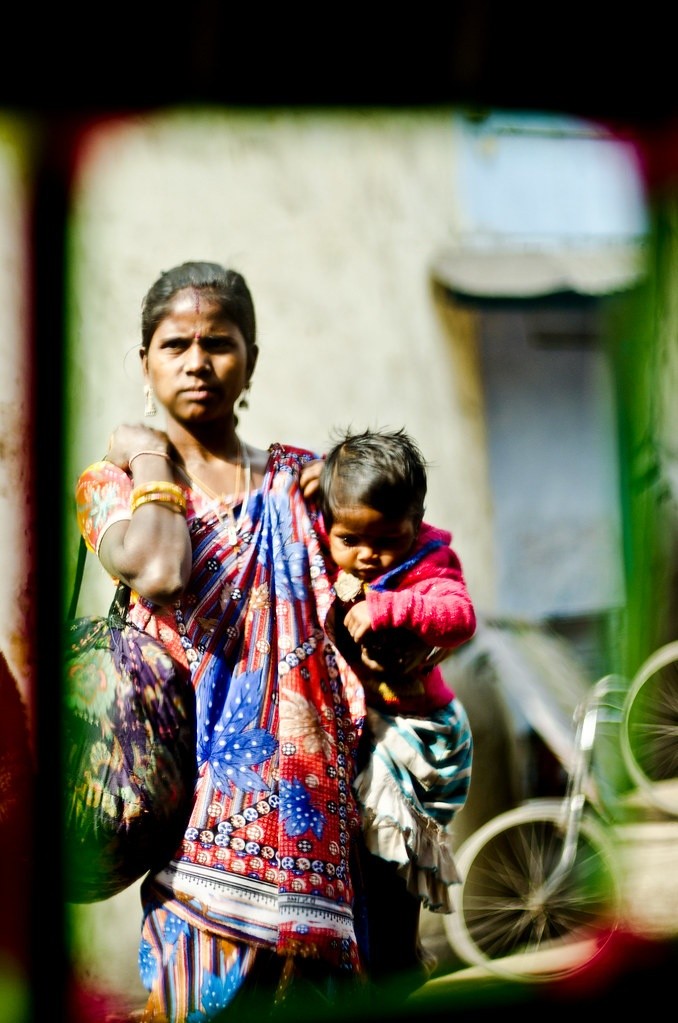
xmin=175 ymin=440 xmax=251 ymax=547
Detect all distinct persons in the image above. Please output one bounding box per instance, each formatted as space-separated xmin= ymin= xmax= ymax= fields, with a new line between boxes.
xmin=300 ymin=426 xmax=476 ymax=914
xmin=75 ymin=261 xmax=476 ymax=1023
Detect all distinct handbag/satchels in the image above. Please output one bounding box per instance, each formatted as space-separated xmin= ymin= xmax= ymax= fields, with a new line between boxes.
xmin=62 ymin=455 xmax=194 ymax=904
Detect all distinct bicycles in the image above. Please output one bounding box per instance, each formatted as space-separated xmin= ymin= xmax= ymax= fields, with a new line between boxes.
xmin=442 ymin=632 xmax=678 ymax=985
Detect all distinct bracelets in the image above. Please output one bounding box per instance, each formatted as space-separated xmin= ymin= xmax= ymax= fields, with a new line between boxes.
xmin=129 ymin=451 xmax=172 ymax=471
xmin=130 ymin=481 xmax=186 ymax=516
